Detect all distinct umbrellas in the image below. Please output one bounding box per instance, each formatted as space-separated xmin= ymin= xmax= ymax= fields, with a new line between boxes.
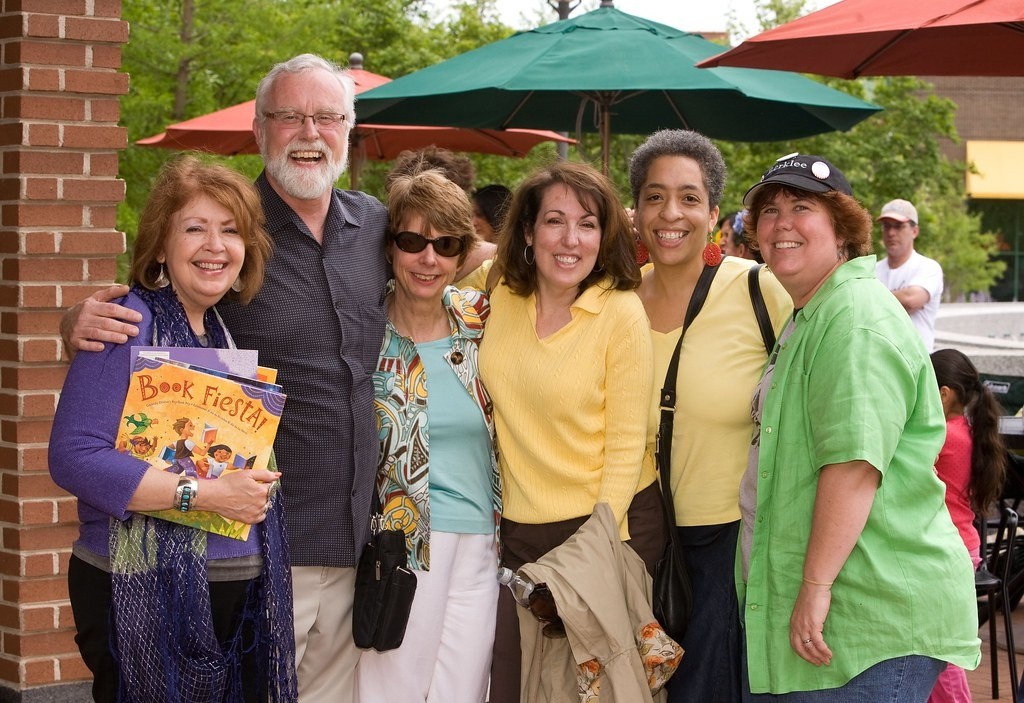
xmin=136 ymin=53 xmax=581 ymax=189
xmin=356 ymin=0 xmax=883 ymax=176
xmin=697 ymin=0 xmax=1024 ymax=79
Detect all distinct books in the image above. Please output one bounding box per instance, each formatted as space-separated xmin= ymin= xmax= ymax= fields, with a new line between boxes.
xmin=115 ymin=346 xmax=287 ymax=541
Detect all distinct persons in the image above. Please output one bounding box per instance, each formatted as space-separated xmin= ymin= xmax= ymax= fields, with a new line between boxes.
xmin=47 ymin=157 xmax=296 ymax=703
xmin=925 ymin=348 xmax=1009 ymax=703
xmin=634 ymin=130 xmax=795 ymax=703
xmin=455 ymin=159 xmax=664 ymax=703
xmin=60 ymin=52 xmax=498 ymax=703
xmin=874 ymin=198 xmax=943 ymax=352
xmin=388 ymin=146 xmax=515 ymax=244
xmin=354 ymin=173 xmax=503 ymax=703
xmin=734 ymin=151 xmax=982 ymax=703
xmin=716 ymin=210 xmax=764 ymax=263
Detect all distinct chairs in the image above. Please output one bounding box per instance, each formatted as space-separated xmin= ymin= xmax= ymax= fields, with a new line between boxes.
xmin=977 ymin=445 xmax=1024 ymax=703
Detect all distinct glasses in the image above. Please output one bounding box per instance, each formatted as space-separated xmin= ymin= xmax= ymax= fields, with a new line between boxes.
xmin=529 ymin=582 xmax=567 ymax=639
xmin=392 ymin=231 xmax=463 ymax=257
xmin=262 ymin=111 xmax=345 ymax=130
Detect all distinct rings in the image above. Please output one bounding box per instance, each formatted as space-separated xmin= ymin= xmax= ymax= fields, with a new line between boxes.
xmin=803 ymin=639 xmax=812 ymax=644
xmin=268 ymin=481 xmax=277 ymax=496
xmin=264 ymin=505 xmax=267 ymax=514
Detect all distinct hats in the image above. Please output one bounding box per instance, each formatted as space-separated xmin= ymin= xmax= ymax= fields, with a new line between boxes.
xmin=876 ymin=199 xmax=919 ymax=225
xmin=742 ymin=151 xmax=853 ymax=209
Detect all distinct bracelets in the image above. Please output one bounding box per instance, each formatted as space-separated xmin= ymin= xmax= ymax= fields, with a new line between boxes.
xmin=174 ymin=475 xmax=199 ymax=512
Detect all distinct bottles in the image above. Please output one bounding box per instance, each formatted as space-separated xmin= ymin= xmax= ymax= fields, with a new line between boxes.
xmin=497 ymin=567 xmax=533 ymax=611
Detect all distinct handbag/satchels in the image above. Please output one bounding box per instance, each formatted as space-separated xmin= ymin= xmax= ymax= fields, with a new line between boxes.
xmin=352 ymin=529 xmax=418 ymax=652
xmin=653 ymin=495 xmax=695 ymax=642
